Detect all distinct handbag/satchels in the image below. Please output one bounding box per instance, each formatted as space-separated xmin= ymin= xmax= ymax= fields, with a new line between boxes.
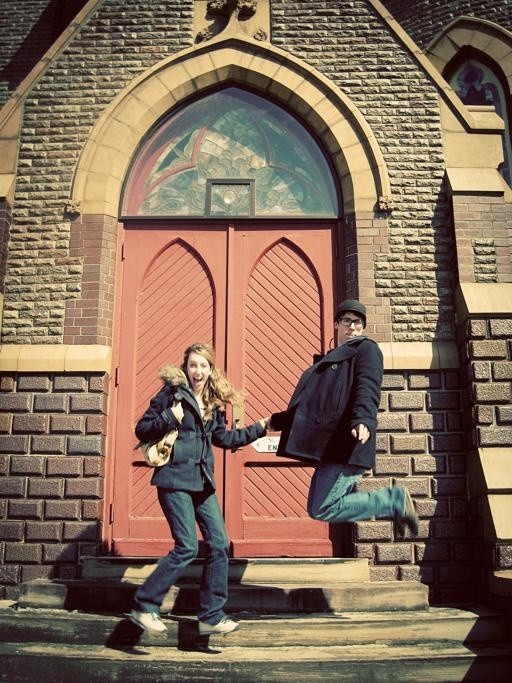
xmin=133 ymin=430 xmax=178 ymax=469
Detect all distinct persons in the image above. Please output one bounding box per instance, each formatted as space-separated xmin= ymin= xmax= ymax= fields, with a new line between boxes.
xmin=123 ymin=340 xmax=273 ymax=635
xmin=265 ymin=297 xmax=420 ymax=544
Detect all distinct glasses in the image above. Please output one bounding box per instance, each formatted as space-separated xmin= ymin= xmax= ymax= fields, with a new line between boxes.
xmin=338 ymin=316 xmax=362 ymax=328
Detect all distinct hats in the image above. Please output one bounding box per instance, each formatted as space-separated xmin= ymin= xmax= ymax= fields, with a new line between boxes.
xmin=333 ymin=298 xmax=367 ymax=328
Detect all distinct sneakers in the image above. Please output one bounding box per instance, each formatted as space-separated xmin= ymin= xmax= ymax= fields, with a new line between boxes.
xmin=127 ymin=607 xmax=168 ymax=635
xmin=394 ymin=488 xmax=422 ymax=540
xmin=197 ymin=615 xmax=242 ymax=635
